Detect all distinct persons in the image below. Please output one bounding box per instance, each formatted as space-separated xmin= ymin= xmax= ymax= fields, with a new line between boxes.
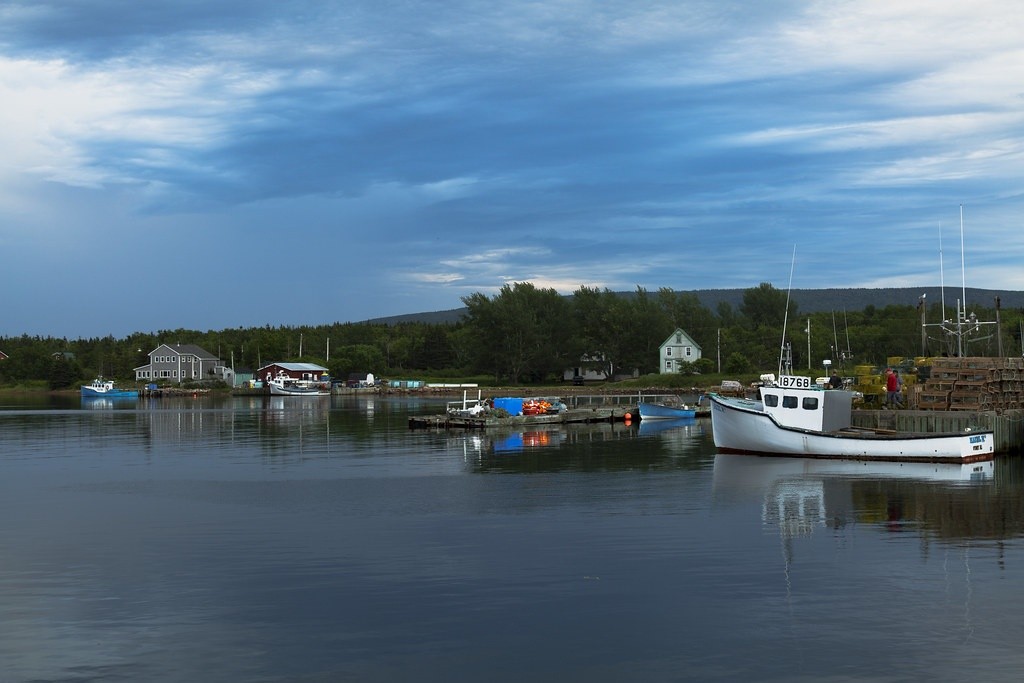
xmin=828 ymin=371 xmax=843 ymax=390
xmin=881 ymin=368 xmax=906 ymax=409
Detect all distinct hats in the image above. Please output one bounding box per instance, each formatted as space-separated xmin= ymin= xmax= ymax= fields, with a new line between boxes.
xmin=885 ymin=369 xmax=891 ymax=373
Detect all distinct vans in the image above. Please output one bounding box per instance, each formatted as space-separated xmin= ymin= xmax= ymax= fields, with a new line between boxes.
xmin=721 ymin=380 xmax=744 ymax=394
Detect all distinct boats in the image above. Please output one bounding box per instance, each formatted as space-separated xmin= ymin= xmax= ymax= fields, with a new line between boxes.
xmin=636 ymin=399 xmax=696 ymax=420
xmin=267 ymin=369 xmax=332 ymax=397
xmin=80 ymin=361 xmax=138 ymax=398
xmin=702 ymin=243 xmax=997 ymax=465
